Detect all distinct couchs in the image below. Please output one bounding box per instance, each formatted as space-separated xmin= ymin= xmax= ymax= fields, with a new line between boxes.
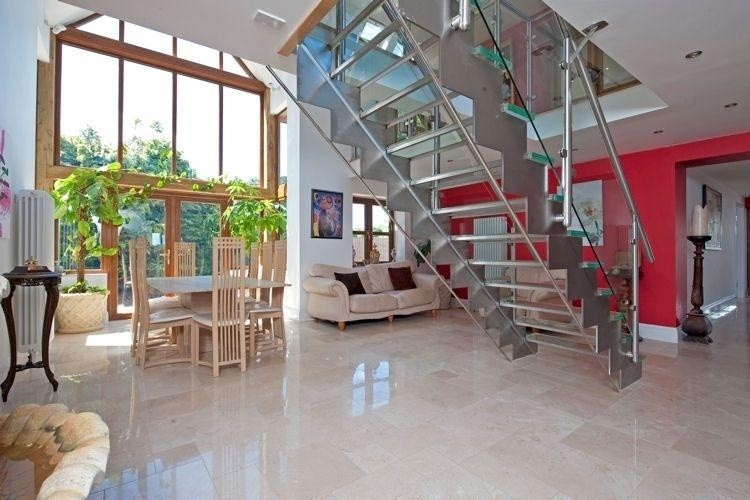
xmin=303 ymin=261 xmax=446 ymax=330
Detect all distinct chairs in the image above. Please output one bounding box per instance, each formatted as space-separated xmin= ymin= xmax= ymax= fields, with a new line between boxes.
xmin=128 ymin=237 xmax=288 ymax=378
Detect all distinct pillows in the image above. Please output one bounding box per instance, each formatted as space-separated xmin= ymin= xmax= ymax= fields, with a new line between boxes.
xmin=358 ymin=269 xmax=393 ymax=294
xmin=387 ymin=266 xmax=418 ymax=291
xmin=334 ymin=271 xmax=366 ymax=294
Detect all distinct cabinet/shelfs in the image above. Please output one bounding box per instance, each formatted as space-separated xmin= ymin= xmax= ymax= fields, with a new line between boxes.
xmin=511 ymin=260 xmax=584 ymax=336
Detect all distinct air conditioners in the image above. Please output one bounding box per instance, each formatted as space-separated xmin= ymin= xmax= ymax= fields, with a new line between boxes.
xmin=49 ymin=159 xmax=156 ymax=332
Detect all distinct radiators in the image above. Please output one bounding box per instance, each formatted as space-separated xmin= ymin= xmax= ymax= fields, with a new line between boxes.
xmin=14 ymin=189 xmax=56 ymax=355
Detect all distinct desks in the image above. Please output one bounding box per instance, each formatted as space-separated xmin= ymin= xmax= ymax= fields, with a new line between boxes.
xmin=0 ymin=272 xmax=64 ymax=400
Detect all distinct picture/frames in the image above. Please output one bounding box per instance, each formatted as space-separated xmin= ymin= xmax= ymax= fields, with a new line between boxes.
xmin=309 ymin=187 xmax=344 ymax=241
xmin=700 ymin=183 xmax=723 ymax=252
